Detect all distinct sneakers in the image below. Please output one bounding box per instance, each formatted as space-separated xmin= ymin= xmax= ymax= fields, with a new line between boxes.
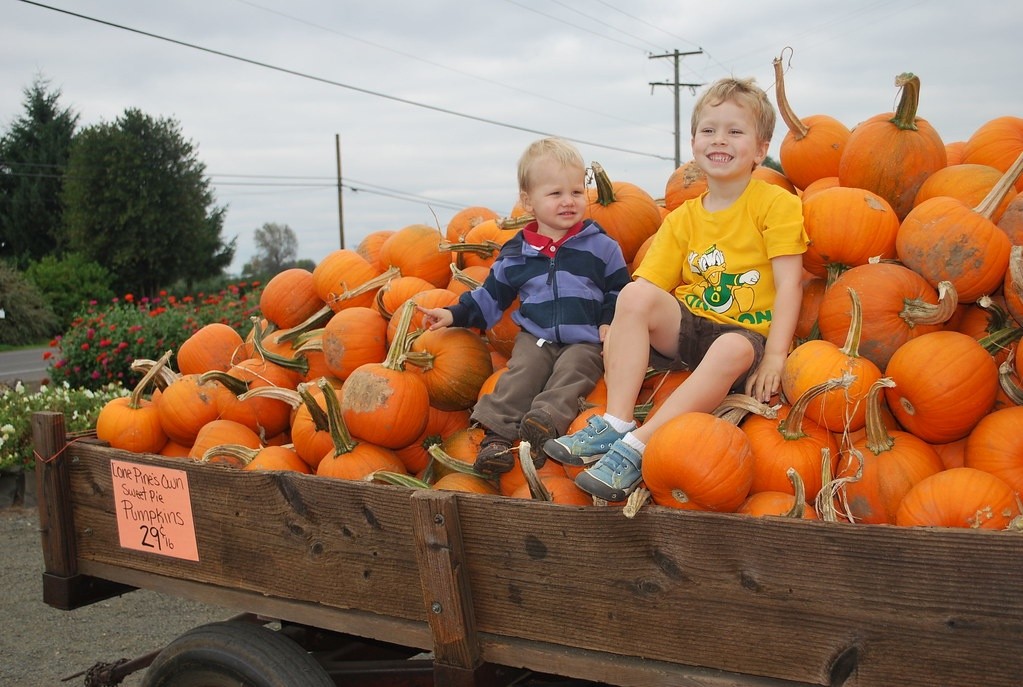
xmin=574 ymin=437 xmax=645 ymax=502
xmin=473 ymin=428 xmax=515 ymax=476
xmin=519 ymin=408 xmax=555 ymax=470
xmin=543 ymin=414 xmax=638 ymax=466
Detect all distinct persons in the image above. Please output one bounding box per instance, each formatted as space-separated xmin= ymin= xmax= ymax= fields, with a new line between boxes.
xmin=414 ymin=138 xmax=631 ymax=480
xmin=543 ymin=78 xmax=809 ymax=503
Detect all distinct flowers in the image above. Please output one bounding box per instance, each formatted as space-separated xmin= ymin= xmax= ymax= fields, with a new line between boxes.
xmin=0 ymin=378 xmax=144 ymax=471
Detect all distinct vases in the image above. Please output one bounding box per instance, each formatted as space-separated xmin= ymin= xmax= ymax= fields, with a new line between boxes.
xmin=16 ymin=467 xmax=42 ymax=508
xmin=0 ymin=464 xmax=24 ymax=510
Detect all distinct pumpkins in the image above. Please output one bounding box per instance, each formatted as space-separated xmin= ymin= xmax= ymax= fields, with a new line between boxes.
xmin=96 ymin=59 xmax=1023 ymax=534
